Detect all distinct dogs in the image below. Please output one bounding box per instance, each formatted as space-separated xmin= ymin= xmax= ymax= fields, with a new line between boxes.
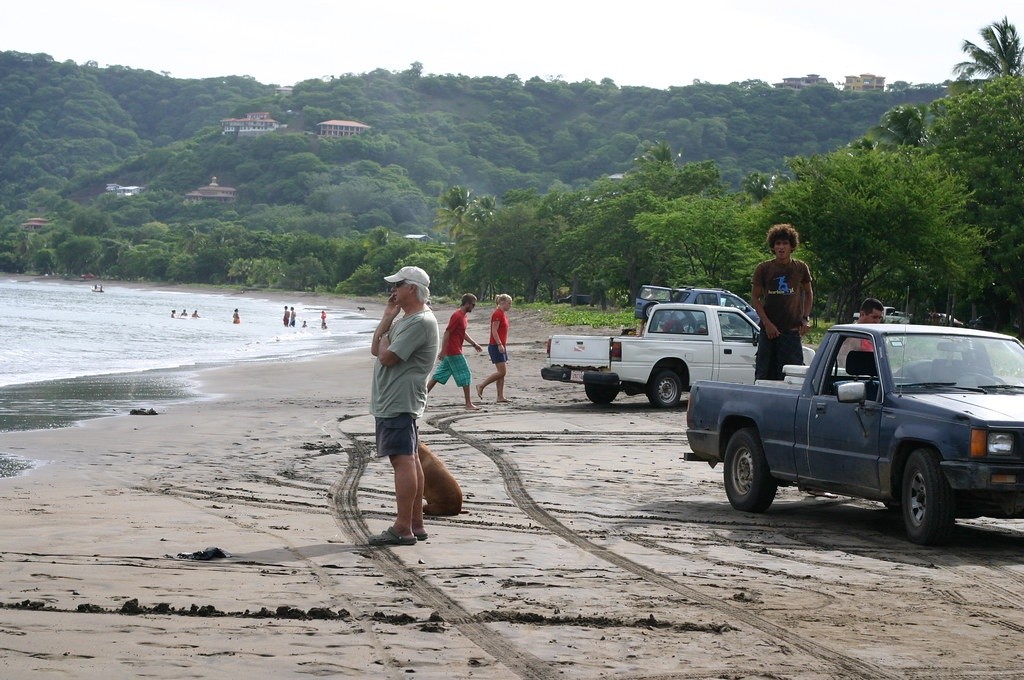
xmin=357 ymin=307 xmax=366 ymax=312
xmin=417 ymin=440 xmax=462 ymax=515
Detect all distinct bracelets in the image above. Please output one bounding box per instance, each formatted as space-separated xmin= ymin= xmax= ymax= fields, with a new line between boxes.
xmin=497 ymin=342 xmax=502 ymax=346
xmin=378 ymin=333 xmax=389 ymax=341
xmin=473 ymin=343 xmax=478 ymax=347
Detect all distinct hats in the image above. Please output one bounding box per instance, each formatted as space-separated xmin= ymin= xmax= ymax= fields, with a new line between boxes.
xmin=384 ymin=266 xmax=430 ymax=286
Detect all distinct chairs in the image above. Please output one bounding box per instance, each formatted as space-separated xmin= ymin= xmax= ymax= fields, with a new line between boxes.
xmin=934 ymin=358 xmax=963 ymax=388
xmin=832 ymin=350 xmax=879 ymax=399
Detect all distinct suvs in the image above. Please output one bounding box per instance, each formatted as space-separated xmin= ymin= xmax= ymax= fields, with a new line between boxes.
xmin=635 ymin=282 xmax=760 ymax=329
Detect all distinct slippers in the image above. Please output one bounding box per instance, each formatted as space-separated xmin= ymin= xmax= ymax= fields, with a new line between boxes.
xmin=382 ymin=531 xmax=428 ymax=540
xmin=367 ymin=526 xmax=417 ymax=544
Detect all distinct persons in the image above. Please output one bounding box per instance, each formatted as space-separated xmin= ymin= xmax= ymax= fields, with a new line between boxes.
xmin=476 ymin=294 xmax=513 ymax=402
xmin=302 ymin=321 xmax=307 ymax=327
xmin=283 ymin=306 xmax=296 ymax=327
xmin=751 ymin=224 xmax=813 ymax=384
xmin=233 ymin=309 xmax=240 ymax=324
xmin=94 ymin=283 xmax=102 ymax=291
xmin=367 ymin=266 xmax=439 ymax=545
xmin=171 ymin=309 xmax=200 ymax=318
xmin=837 ymin=298 xmax=884 ymax=368
xmin=321 ymin=310 xmax=326 ymax=328
xmin=427 ymin=293 xmax=482 ymax=410
xmin=663 ymin=311 xmax=684 ymax=333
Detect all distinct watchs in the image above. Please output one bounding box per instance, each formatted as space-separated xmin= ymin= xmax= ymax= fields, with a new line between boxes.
xmin=802 ymin=315 xmax=810 ymax=321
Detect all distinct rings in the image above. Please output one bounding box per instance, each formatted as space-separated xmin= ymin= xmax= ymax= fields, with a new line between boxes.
xmin=438 ymin=356 xmax=441 ymax=359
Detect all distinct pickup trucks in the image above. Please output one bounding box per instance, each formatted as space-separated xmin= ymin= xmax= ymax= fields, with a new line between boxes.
xmin=541 ymin=302 xmax=816 ymax=408
xmin=683 ymin=323 xmax=1024 ymax=546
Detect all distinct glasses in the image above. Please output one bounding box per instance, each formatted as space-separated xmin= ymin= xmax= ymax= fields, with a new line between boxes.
xmin=394 ymin=282 xmax=407 ymax=287
xmin=471 ymin=303 xmax=475 ymax=308
